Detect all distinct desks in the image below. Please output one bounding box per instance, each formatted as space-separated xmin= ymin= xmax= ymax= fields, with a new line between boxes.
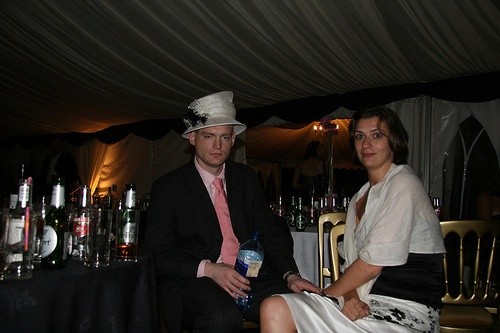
xmin=291 ymin=231 xmax=331 ymax=288
xmin=0 ymin=245 xmax=168 ymax=333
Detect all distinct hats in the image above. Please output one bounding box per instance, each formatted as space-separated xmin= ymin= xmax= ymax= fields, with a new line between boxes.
xmin=182 ymin=91 xmax=247 ymax=140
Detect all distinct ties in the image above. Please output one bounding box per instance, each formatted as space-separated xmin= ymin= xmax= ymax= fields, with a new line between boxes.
xmin=213 ymin=178 xmax=239 ymax=269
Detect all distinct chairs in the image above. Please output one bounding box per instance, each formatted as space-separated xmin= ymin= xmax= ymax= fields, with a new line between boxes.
xmin=318 ymin=213 xmax=500 ymax=333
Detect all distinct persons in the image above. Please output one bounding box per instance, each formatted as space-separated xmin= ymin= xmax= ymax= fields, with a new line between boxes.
xmin=145 ymin=91 xmax=323 ymax=333
xmin=259 ymin=106 xmax=447 ymax=333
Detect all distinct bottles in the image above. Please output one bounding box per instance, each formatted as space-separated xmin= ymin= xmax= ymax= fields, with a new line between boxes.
xmin=231 ymin=233 xmax=264 ymax=304
xmin=269 ymin=185 xmax=351 ymax=230
xmin=432 ymin=196 xmax=445 ymax=221
xmin=0 ymin=162 xmax=148 ymax=280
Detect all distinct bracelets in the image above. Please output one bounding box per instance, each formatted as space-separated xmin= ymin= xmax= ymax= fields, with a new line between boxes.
xmin=285 ymin=272 xmax=302 ymax=282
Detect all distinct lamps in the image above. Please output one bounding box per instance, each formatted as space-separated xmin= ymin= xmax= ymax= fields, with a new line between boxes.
xmin=314 ymin=121 xmax=339 ymax=138
